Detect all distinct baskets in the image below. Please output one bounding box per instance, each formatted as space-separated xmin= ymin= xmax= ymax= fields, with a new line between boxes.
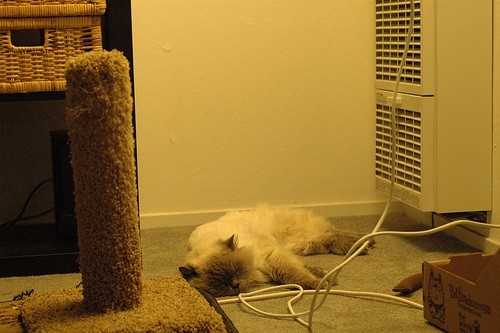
xmin=0 ymin=0 xmax=107 ymax=94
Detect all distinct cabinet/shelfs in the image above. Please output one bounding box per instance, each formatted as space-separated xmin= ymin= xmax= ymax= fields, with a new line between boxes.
xmin=0 ymin=0 xmax=138 ymax=278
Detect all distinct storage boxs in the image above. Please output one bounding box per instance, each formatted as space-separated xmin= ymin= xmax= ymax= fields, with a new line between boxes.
xmin=423 ymin=245 xmax=500 ymax=333
xmin=0 ymin=0 xmax=108 ymax=93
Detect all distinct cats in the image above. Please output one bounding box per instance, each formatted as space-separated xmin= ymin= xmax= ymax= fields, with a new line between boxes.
xmin=179 ymin=205 xmax=376 ymax=298
